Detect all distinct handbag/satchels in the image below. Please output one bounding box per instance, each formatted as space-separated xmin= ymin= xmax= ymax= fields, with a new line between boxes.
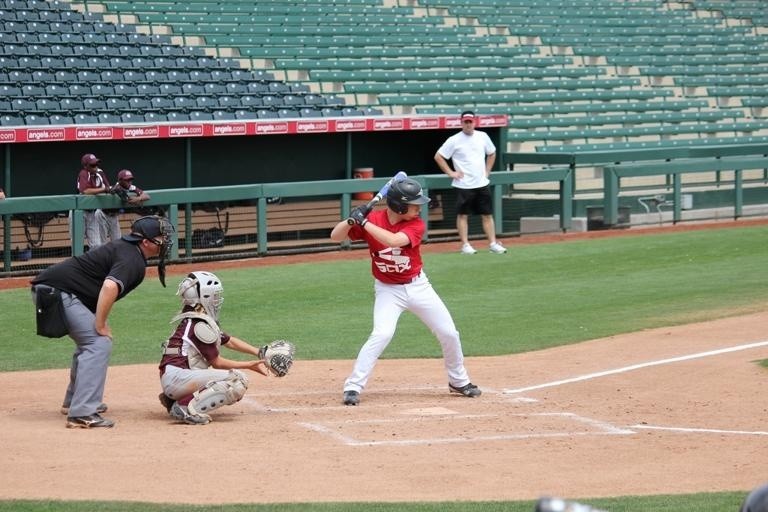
xmin=193 ymin=227 xmax=225 ymax=249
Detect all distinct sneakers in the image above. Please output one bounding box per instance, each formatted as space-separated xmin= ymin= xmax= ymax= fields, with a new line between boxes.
xmin=461 ymin=243 xmax=477 ymax=255
xmin=448 ymin=382 xmax=481 ymax=397
xmin=159 ymin=392 xmax=175 ymax=413
xmin=343 ymin=390 xmax=360 ymax=406
xmin=169 ymin=401 xmax=211 ymax=425
xmin=489 ymin=242 xmax=507 ymax=255
xmin=60 ymin=401 xmax=107 ymax=415
xmin=66 ymin=412 xmax=115 ymax=430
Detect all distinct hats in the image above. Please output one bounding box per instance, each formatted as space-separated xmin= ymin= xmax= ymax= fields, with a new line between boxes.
xmin=461 ymin=110 xmax=475 ymax=123
xmin=122 ymin=216 xmax=163 ymax=243
xmin=117 ymin=169 xmax=134 ymax=181
xmin=81 ymin=153 xmax=100 ymax=166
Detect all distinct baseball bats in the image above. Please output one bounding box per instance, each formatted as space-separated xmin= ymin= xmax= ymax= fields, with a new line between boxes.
xmin=348 ymin=171 xmax=408 ymax=225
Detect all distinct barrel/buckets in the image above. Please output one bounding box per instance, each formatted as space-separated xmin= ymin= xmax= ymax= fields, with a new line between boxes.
xmin=352 ymin=165 xmax=373 ymax=203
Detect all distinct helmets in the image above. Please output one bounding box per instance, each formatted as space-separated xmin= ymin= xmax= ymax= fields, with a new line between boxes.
xmin=176 ymin=271 xmax=224 ymax=308
xmin=386 ymin=179 xmax=431 ymax=215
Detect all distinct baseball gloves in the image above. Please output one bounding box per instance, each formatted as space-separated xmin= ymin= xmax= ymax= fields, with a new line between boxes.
xmin=258 ymin=340 xmax=295 ymax=377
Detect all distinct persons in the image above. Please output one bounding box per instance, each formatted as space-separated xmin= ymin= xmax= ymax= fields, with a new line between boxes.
xmin=68 ymin=153 xmax=110 ymax=257
xmin=94 ymin=168 xmax=151 ymax=245
xmin=327 ymin=177 xmax=482 ymax=407
xmin=157 ymin=268 xmax=297 ymax=427
xmin=432 ymin=110 xmax=509 ymax=255
xmin=28 ymin=215 xmax=177 ymax=429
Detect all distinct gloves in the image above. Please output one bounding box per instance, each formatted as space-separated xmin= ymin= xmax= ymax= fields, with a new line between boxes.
xmin=357 ymin=205 xmax=373 ymax=217
xmin=349 ymin=207 xmax=369 ymax=227
